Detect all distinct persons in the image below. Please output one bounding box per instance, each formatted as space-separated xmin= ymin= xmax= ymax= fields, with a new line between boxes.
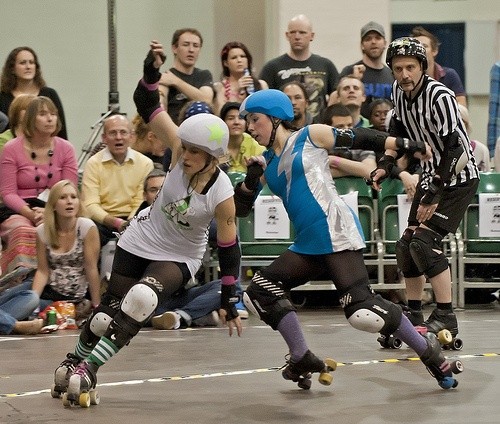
xmin=0 ymin=15 xmax=500 ymax=408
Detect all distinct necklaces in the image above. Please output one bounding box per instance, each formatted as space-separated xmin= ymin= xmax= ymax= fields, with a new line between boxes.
xmin=27 ymin=136 xmax=53 ymax=196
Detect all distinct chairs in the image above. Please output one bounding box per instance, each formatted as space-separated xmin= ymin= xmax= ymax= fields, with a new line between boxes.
xmin=196 ymin=168 xmax=499 ymax=309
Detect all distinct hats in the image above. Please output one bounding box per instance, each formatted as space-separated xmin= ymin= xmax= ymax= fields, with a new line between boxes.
xmin=360 ymin=21 xmax=386 ymax=40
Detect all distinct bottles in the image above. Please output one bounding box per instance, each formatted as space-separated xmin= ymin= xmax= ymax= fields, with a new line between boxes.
xmin=243 ymin=68 xmax=255 ymax=96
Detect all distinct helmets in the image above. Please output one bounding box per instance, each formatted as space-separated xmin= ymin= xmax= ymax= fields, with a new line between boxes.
xmin=177 ymin=114 xmax=230 ymax=157
xmin=386 ymin=37 xmax=429 ymax=70
xmin=239 ymin=89 xmax=294 ymax=121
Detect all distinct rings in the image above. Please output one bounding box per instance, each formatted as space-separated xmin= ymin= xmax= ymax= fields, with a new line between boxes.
xmin=427 ymin=210 xmax=431 ymax=213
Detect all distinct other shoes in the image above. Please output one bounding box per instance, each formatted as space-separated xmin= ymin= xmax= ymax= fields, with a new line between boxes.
xmin=236 ymin=293 xmax=249 ymax=319
xmin=193 ymin=311 xmax=219 ymax=326
xmin=16 ymin=318 xmax=43 ymax=335
xmin=152 ymin=310 xmax=180 ymax=330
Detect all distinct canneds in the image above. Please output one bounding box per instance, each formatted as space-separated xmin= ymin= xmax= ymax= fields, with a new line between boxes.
xmin=46 ymin=310 xmax=56 ymax=326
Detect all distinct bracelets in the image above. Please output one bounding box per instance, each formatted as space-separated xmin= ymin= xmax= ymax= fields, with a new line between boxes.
xmin=333 ymin=157 xmax=341 ymax=167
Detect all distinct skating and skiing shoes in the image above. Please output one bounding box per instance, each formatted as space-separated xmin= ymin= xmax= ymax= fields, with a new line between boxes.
xmin=280 ymin=349 xmax=338 ymax=390
xmin=62 ymin=363 xmax=101 ymax=409
xmin=50 ymin=356 xmax=82 ymax=398
xmin=375 ymin=309 xmax=427 ymax=348
xmin=414 ymin=310 xmax=463 ymax=351
xmin=421 ymin=329 xmax=465 ymax=389
